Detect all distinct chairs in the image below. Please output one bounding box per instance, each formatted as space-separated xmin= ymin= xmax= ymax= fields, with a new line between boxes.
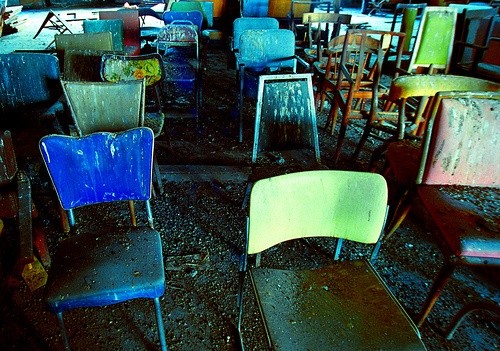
xmin=0 ymin=0 xmax=500 ymax=351
